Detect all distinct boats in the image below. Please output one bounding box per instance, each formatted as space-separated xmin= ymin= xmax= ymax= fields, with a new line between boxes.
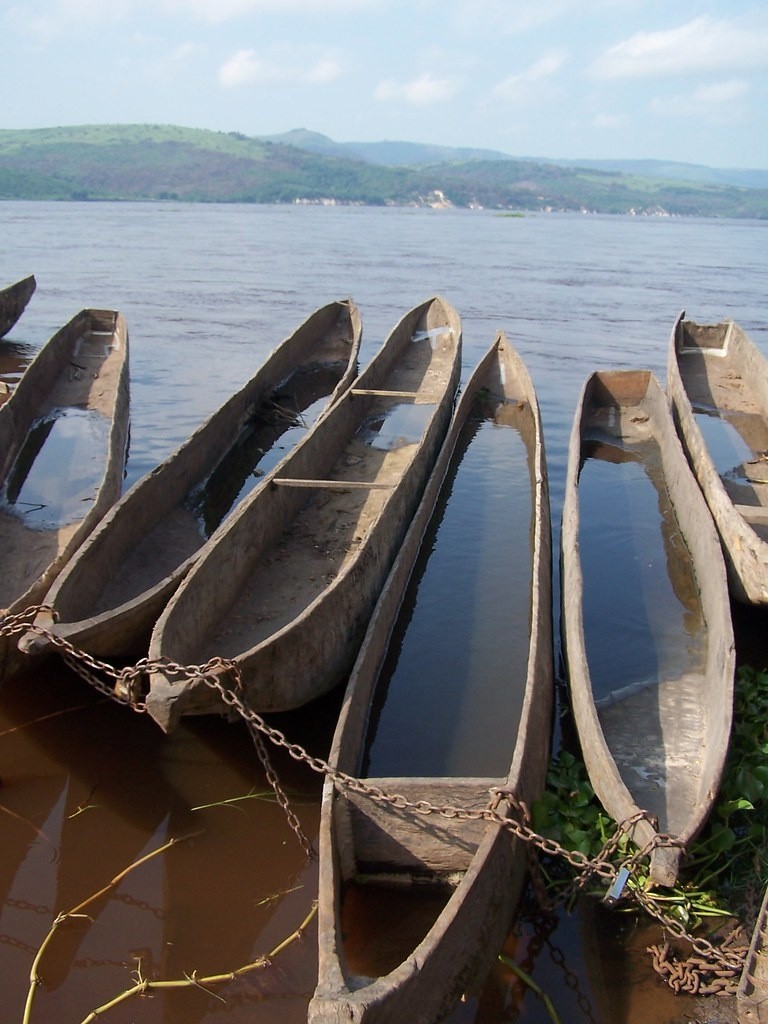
xmin=143 ymin=291 xmax=465 ymax=737
xmin=13 ymin=296 xmax=363 ymax=661
xmin=301 ymin=330 xmax=551 ymax=1024
xmin=664 ymin=308 xmax=768 ymax=608
xmin=0 ymin=275 xmax=38 ymax=340
xmin=559 ymin=364 xmax=737 ymax=892
xmin=0 ymin=308 xmax=133 ymax=626
